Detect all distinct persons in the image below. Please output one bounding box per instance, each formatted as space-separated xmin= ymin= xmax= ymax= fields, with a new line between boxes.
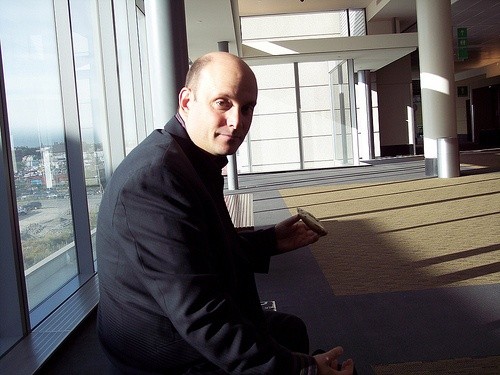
xmin=95 ymin=51 xmax=360 ymax=375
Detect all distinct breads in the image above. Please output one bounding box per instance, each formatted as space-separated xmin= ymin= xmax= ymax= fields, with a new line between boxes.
xmin=297 ymin=206 xmax=328 ymax=237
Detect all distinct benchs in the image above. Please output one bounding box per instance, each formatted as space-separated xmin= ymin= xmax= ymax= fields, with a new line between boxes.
xmin=224 ymin=193 xmax=255 ymax=232
